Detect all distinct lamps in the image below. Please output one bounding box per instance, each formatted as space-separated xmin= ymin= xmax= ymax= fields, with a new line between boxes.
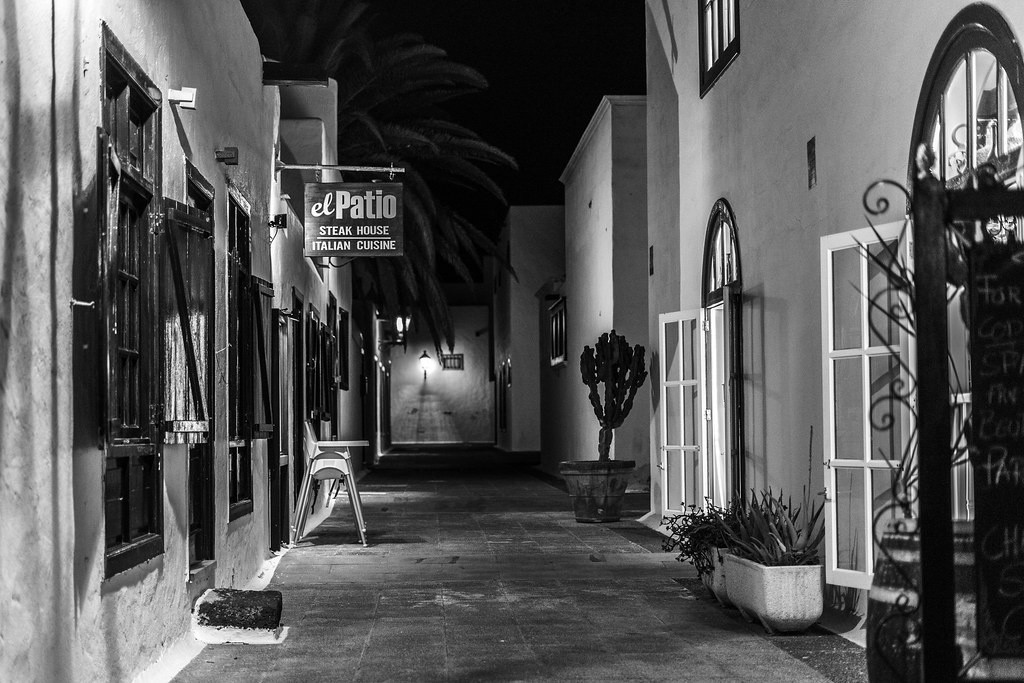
xmin=215 ymin=147 xmax=239 ymax=166
xmin=419 ymin=349 xmax=432 ymax=379
xmin=269 ymin=214 xmax=287 ymax=229
xmin=378 ymin=303 xmax=413 ymax=354
xmin=168 ymin=87 xmax=198 ymax=111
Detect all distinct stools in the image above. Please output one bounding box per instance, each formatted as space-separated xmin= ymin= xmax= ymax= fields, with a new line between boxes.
xmin=291 ymin=421 xmax=371 ymax=548
xmin=300 ymin=421 xmax=368 ymax=537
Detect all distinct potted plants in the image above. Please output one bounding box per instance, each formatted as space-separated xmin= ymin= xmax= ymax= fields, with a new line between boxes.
xmin=843 ymin=212 xmax=973 ymax=683
xmin=712 ymin=484 xmax=825 ymax=634
xmin=659 ymin=495 xmax=790 ymax=601
xmin=555 ymin=329 xmax=648 ymax=524
